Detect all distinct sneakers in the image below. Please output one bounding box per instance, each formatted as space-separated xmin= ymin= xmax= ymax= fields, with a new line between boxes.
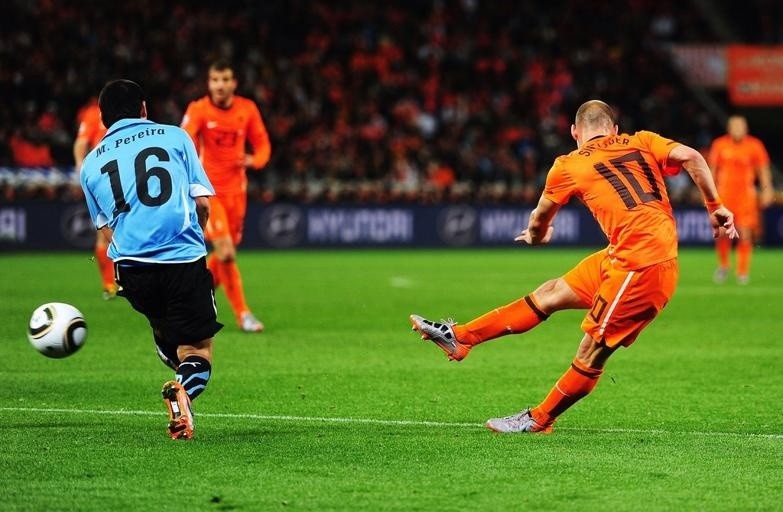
xmin=486 ymin=410 xmax=552 ymax=433
xmin=242 ymin=316 xmax=263 ymax=332
xmin=162 ymin=381 xmax=194 ymax=439
xmin=410 ymin=314 xmax=472 ymax=361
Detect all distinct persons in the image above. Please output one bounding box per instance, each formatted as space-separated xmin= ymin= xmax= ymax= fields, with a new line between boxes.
xmin=79 ymin=78 xmax=223 ymax=438
xmin=1 ymin=1 xmax=727 ymax=203
xmin=71 ymin=78 xmax=119 ymax=300
xmin=710 ymin=115 xmax=776 ymax=285
xmin=179 ymin=58 xmax=272 ymax=332
xmin=407 ymin=99 xmax=738 ymax=433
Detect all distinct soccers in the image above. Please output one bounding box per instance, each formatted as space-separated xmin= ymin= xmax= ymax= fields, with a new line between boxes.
xmin=28 ymin=301 xmax=88 ymax=359
